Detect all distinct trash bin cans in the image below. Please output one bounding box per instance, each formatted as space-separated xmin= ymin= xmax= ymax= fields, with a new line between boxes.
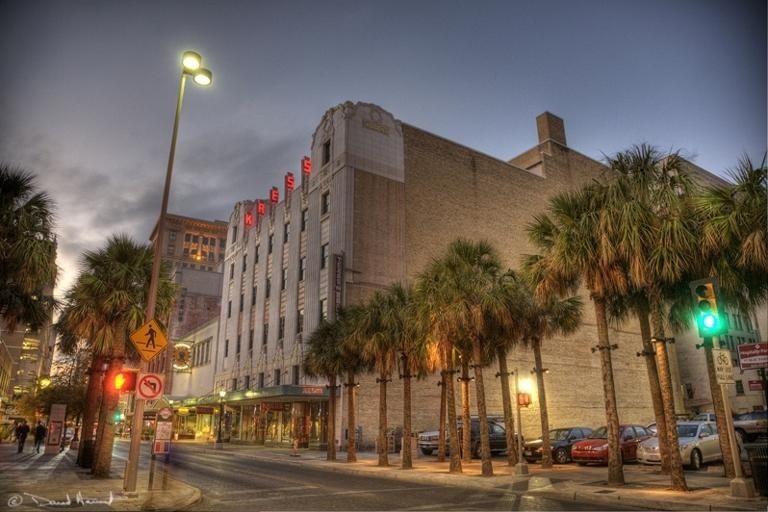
xmin=81 ymin=439 xmax=96 ymax=468
xmin=744 ymin=443 xmax=768 ymax=496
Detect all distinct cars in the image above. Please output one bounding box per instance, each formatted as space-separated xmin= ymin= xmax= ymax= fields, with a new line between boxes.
xmin=524 ymin=427 xmax=597 ymax=465
xmin=694 ymin=413 xmax=717 ymax=421
xmin=636 ymin=421 xmax=742 ymax=471
xmin=418 ymin=417 xmax=525 ymax=459
xmin=734 ymin=410 xmax=767 ymax=443
xmin=573 ymin=423 xmax=657 ymax=467
xmin=60 ymin=429 xmax=75 ymax=446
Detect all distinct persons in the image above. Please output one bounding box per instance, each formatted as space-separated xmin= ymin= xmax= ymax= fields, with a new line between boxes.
xmin=14 ymin=421 xmax=29 ymax=454
xmin=32 ymin=420 xmax=47 ymax=453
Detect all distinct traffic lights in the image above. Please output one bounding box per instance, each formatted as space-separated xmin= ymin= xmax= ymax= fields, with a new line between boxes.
xmin=112 ymin=370 xmax=136 ymax=391
xmin=689 ymin=277 xmax=722 ymax=339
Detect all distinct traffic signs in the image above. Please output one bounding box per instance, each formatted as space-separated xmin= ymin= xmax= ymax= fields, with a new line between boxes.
xmin=738 ymin=342 xmax=768 ymax=371
xmin=711 ymin=347 xmax=738 ymax=384
xmin=748 ymin=379 xmax=763 ymax=391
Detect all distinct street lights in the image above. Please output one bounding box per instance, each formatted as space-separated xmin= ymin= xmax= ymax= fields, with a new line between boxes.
xmin=118 ymin=48 xmax=212 ymax=494
xmin=514 ymin=368 xmax=534 ymax=478
xmin=215 ymin=383 xmax=226 ymax=449
